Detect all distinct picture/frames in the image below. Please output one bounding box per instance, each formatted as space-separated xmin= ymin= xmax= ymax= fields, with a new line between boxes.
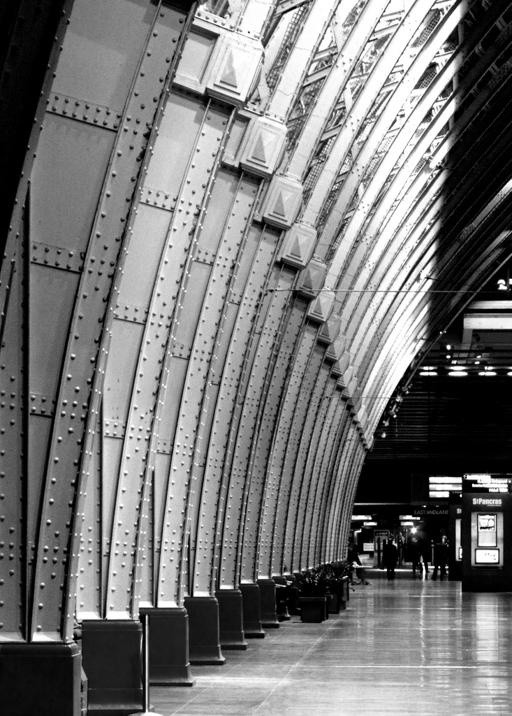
xmin=475 ymin=549 xmax=500 ymax=564
xmin=478 ymin=513 xmax=497 ymax=546
xmin=459 ymin=547 xmax=463 ymax=558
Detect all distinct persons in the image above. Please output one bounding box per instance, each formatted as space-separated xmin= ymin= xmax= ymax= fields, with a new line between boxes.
xmin=345 ymin=527 xmax=454 ymax=586
